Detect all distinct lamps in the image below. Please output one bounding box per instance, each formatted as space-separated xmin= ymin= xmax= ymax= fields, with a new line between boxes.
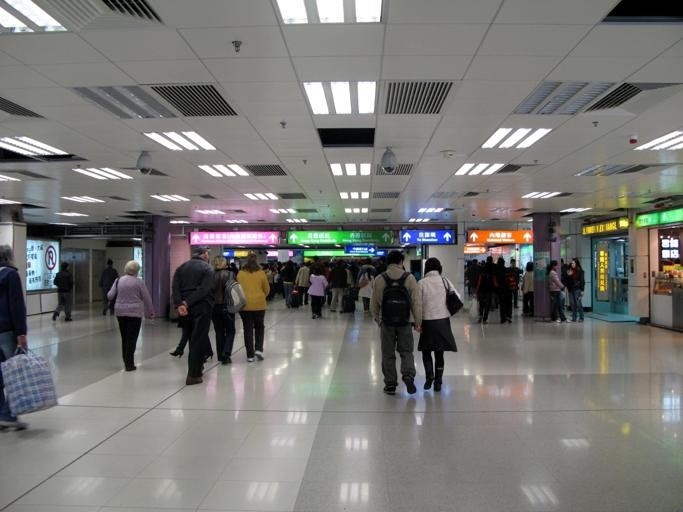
xmin=381 ymin=146 xmax=399 ymax=174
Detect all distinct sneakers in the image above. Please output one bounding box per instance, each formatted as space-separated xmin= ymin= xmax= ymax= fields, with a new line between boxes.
xmin=383 ymin=384 xmax=397 ymax=395
xmin=404 ymin=375 xmax=417 ymax=394
xmin=218 ymin=355 xmax=233 ymax=365
xmin=311 ymin=312 xmax=322 ymax=319
xmin=248 ymin=356 xmax=255 ymax=362
xmin=552 ymin=316 xmax=585 ymax=324
xmin=478 ymin=317 xmax=488 ymax=324
xmin=186 ymin=367 xmax=204 ymax=385
xmin=254 ymin=350 xmax=265 ymax=360
xmin=103 ymin=305 xmax=114 ymax=316
xmin=0 ymin=419 xmax=29 ymax=433
xmin=52 ymin=312 xmax=73 ymax=322
xmin=501 ymin=317 xmax=513 ymax=324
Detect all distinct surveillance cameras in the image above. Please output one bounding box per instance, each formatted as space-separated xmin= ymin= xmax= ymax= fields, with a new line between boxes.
xmin=380 ymin=149 xmax=398 ymax=174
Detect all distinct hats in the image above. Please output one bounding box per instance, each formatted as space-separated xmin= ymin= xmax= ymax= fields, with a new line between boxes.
xmin=106 ymin=259 xmax=113 ymax=265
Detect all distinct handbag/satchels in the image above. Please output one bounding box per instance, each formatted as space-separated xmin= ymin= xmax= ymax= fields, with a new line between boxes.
xmin=441 ymin=276 xmax=464 ymax=318
xmin=109 ymin=278 xmax=119 ymax=315
xmin=358 ymin=273 xmax=369 ymax=288
xmin=1 ymin=345 xmax=58 ymax=418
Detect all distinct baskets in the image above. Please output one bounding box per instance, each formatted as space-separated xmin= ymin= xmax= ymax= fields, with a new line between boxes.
xmin=291 ymin=286 xmax=299 ymax=297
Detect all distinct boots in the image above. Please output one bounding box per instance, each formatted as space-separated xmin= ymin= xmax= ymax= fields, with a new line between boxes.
xmin=424 ymin=368 xmax=434 ymax=390
xmin=433 ymin=368 xmax=444 ymax=392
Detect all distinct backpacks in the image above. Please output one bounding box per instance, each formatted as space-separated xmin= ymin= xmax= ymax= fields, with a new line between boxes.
xmin=223 ymin=271 xmax=246 ymax=315
xmin=379 ymin=270 xmax=412 ymax=327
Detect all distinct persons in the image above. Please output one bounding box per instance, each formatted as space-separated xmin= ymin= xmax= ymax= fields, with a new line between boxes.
xmin=465 ymin=256 xmax=585 ymax=325
xmin=52 ymin=262 xmax=74 ymax=322
xmin=106 ymin=261 xmax=155 ymax=371
xmin=168 ymin=248 xmax=270 ymax=386
xmin=100 ymin=257 xmax=119 ymax=316
xmin=0 ymin=245 xmax=28 ymax=430
xmin=370 ymin=249 xmax=461 ymax=394
xmin=257 ymin=256 xmax=387 ymax=319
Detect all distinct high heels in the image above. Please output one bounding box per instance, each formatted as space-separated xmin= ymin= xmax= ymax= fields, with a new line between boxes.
xmin=170 ymin=347 xmax=184 ymax=359
xmin=204 ymin=350 xmax=214 ymax=360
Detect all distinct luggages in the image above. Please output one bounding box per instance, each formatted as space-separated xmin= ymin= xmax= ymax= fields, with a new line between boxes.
xmin=341 ymin=293 xmax=356 ymax=314
